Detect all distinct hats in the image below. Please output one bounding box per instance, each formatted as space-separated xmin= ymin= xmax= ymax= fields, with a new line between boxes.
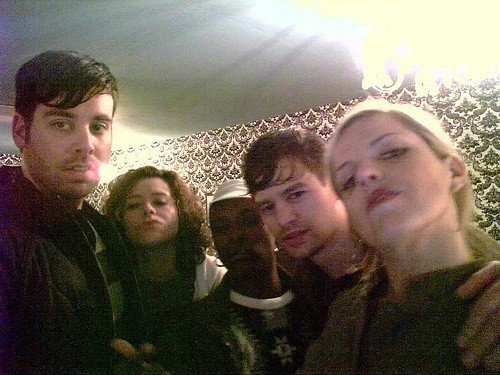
xmin=209 ymin=179 xmax=253 ymax=203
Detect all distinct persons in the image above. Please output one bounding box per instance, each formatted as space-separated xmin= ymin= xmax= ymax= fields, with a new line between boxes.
xmin=109 ymin=180 xmax=323 ymax=375
xmin=0 ymin=48 xmax=148 ymax=375
xmin=99 ymin=165 xmax=229 ymax=336
xmin=297 ymin=99 xmax=500 ymax=375
xmin=242 ymin=129 xmax=500 ymax=375
